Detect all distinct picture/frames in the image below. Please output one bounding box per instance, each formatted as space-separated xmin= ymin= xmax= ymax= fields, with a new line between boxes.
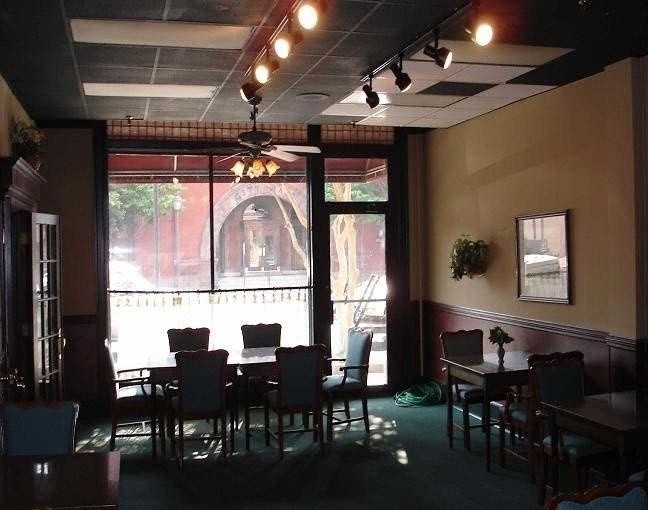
xmin=513 ymin=207 xmax=573 ymax=305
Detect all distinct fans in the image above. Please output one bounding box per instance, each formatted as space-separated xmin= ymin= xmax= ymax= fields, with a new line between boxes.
xmin=200 ymin=96 xmax=321 ymax=163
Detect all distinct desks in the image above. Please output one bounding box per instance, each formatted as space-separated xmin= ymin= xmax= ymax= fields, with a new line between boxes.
xmin=0 ymin=451 xmax=122 ymax=510
xmin=440 ymin=348 xmax=541 ymax=472
xmin=537 ymin=389 xmax=647 ymax=508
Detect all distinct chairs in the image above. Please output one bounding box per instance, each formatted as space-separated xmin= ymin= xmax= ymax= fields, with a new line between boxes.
xmin=543 ymin=480 xmax=647 ymax=509
xmin=238 ymin=323 xmax=282 ymax=433
xmin=317 ymin=327 xmax=374 ymax=442
xmin=147 ymin=346 xmax=324 ymax=457
xmin=499 ymin=349 xmax=585 ymax=484
xmin=530 ymin=358 xmax=615 ymax=509
xmin=168 ymin=328 xmax=210 ymax=434
xmin=103 ymin=337 xmax=170 ymax=459
xmin=264 ymin=343 xmax=327 ymax=458
xmin=440 ymin=327 xmax=514 ymax=451
xmin=0 ymin=400 xmax=81 ymax=459
xmin=171 ymin=350 xmax=230 ymax=466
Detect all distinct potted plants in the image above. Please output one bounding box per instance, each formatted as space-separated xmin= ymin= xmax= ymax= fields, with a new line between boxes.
xmin=447 ymin=233 xmax=489 ymax=285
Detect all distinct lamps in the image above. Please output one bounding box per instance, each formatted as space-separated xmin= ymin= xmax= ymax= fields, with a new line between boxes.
xmin=362 ymin=11 xmax=494 ymax=110
xmin=230 ymin=151 xmax=280 ymax=179
xmin=236 ymin=0 xmax=330 ymax=102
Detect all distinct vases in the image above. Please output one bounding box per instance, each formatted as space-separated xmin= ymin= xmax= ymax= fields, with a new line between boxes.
xmin=15 ymin=146 xmax=41 ymax=172
xmin=496 ymin=344 xmax=505 ymax=370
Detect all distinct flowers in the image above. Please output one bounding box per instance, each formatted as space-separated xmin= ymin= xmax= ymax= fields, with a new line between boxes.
xmin=489 ymin=326 xmax=514 ymax=346
xmin=9 ymin=118 xmax=49 ymax=156
